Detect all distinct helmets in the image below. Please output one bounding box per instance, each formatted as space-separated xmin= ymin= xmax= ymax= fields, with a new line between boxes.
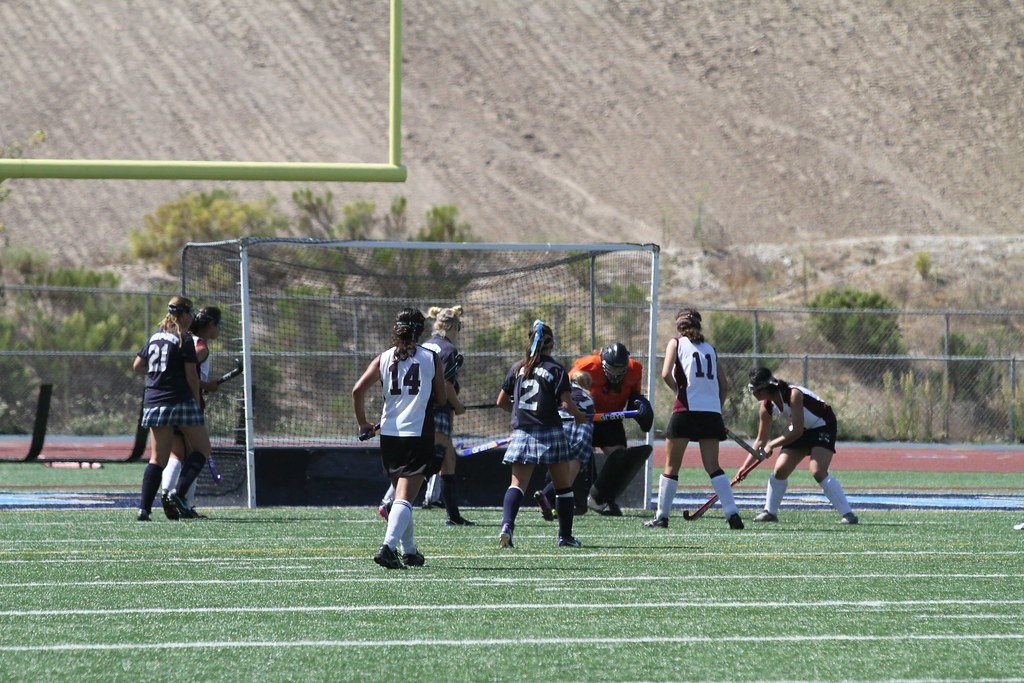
xmin=600 ymin=341 xmax=629 ymax=385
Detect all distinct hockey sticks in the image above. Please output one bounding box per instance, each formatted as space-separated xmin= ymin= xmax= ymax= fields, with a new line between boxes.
xmin=359 ymin=354 xmax=464 ymax=442
xmin=204 ymin=359 xmax=243 ymax=394
xmin=207 ymin=452 xmax=226 ymax=487
xmin=725 ymin=427 xmax=767 ymax=460
xmin=464 ymin=397 xmax=514 ymax=409
xmin=683 ymin=452 xmax=772 ymax=521
xmin=455 ymin=436 xmax=511 ymax=457
xmin=561 ymin=410 xmax=641 ymax=422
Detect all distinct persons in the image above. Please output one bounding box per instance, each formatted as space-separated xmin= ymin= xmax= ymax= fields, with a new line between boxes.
xmin=643 ymin=308 xmax=745 ymax=530
xmin=496 ymin=319 xmax=586 ymax=548
xmin=376 ymin=304 xmax=474 ymax=525
xmin=352 ymin=308 xmax=445 ymax=571
xmin=533 ymin=370 xmax=596 ymax=523
xmin=161 ymin=307 xmax=222 ymax=519
xmin=568 ymin=341 xmax=653 ymax=517
xmin=133 ymin=295 xmax=211 ymax=522
xmin=733 ymin=368 xmax=859 ymax=524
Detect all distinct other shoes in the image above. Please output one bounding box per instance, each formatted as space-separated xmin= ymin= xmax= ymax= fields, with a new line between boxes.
xmin=422 ymin=499 xmax=445 ymax=509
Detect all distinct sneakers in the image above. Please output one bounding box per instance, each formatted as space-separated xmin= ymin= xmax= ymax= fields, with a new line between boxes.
xmin=558 ymin=535 xmax=582 ymax=548
xmin=727 ymin=513 xmax=744 ymax=529
xmin=403 ymin=547 xmax=425 ymax=567
xmin=166 ymin=488 xmax=198 ymax=519
xmin=379 ymin=499 xmax=392 ymax=521
xmin=533 ymin=490 xmax=554 ymax=521
xmin=644 ymin=516 xmax=669 ymax=528
xmin=753 ymin=510 xmax=779 ymax=522
xmin=161 ymin=488 xmax=180 ymax=521
xmin=445 ymin=516 xmax=476 ymax=526
xmin=837 ymin=514 xmax=859 ymax=525
xmin=137 ymin=508 xmax=150 ymax=521
xmin=373 ymin=543 xmax=407 ymax=570
xmin=500 ymin=522 xmax=515 ymax=548
